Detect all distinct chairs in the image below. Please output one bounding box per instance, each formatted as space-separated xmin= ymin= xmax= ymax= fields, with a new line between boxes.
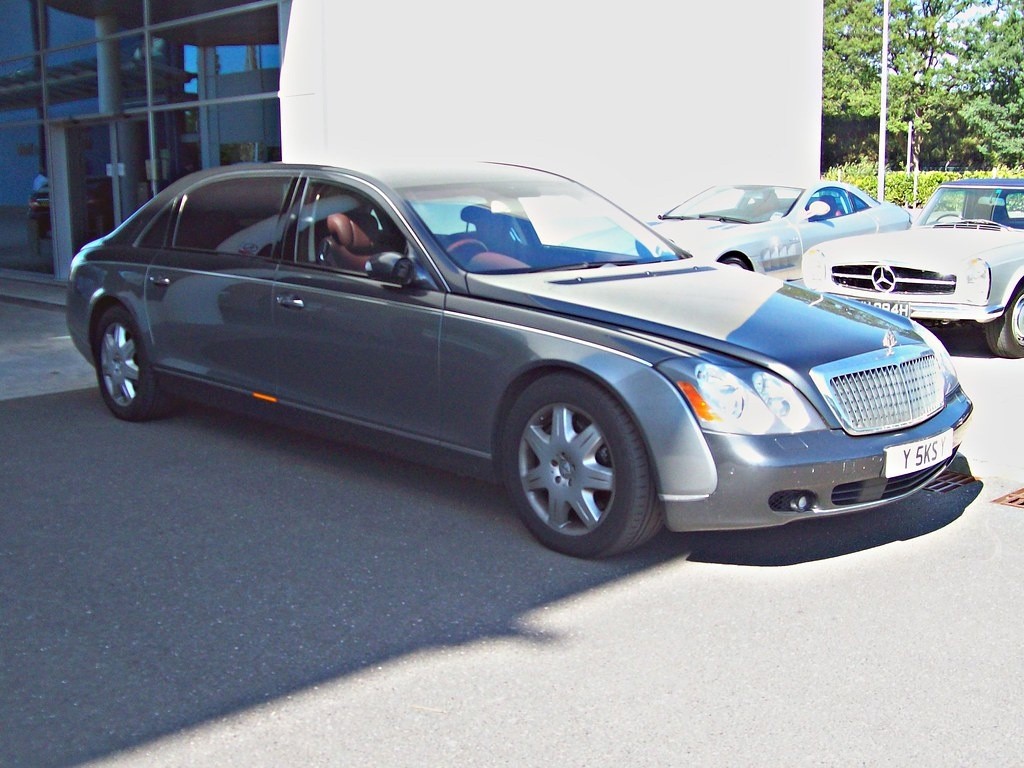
xmin=322 ymin=209 xmax=397 ymax=274
xmin=819 ymin=195 xmax=838 ymax=218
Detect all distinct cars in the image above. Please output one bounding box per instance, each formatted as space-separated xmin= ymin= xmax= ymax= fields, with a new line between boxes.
xmin=643 ymin=180 xmax=912 ymax=281
xmin=800 ymin=179 xmax=1024 ymax=359
xmin=65 ymin=157 xmax=972 ymax=559
